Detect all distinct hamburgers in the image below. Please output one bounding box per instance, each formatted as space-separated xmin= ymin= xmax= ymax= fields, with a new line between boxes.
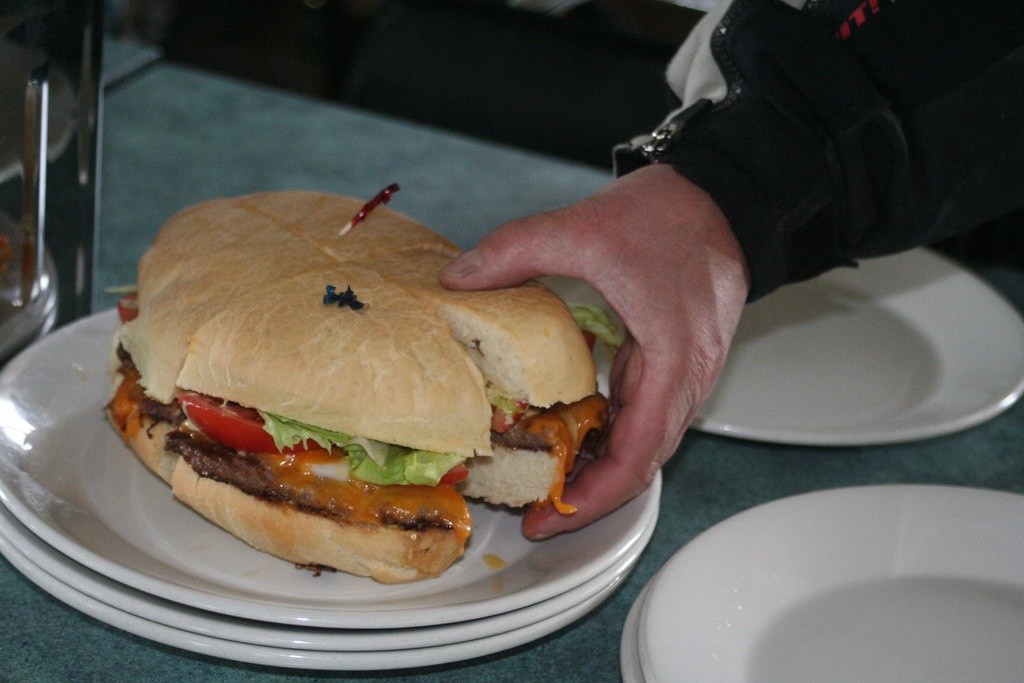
xmin=103 ymin=180 xmax=625 ymax=593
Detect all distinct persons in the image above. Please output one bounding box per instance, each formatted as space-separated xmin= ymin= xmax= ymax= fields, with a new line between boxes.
xmin=435 ymin=1 xmax=1024 ymax=542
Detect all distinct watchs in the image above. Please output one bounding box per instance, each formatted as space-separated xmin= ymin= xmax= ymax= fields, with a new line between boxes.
xmin=638 ymin=98 xmax=835 ymax=305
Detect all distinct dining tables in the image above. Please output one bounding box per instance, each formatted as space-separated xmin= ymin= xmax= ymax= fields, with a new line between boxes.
xmin=0 ymin=38 xmax=1023 ymax=682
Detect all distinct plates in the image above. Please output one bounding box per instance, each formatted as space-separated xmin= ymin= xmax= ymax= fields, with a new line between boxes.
xmin=0 ymin=310 xmax=659 ymax=676
xmin=536 ymin=224 xmax=1024 ymax=445
xmin=619 ymin=486 xmax=1022 ymax=682
